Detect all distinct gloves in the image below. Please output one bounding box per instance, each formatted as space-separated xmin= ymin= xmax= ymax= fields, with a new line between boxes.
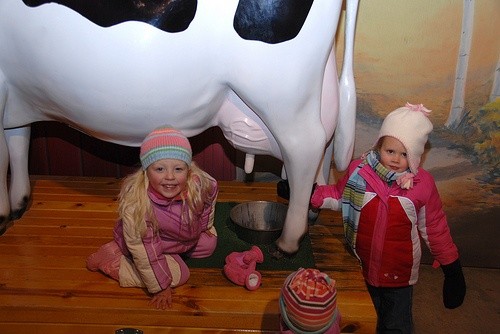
xmin=440 ymin=259 xmax=466 ymax=308
xmin=277 ymin=179 xmax=317 ymax=201
xmin=225 ymin=261 xmax=262 ymax=291
xmin=225 ymin=245 xmax=263 ymax=269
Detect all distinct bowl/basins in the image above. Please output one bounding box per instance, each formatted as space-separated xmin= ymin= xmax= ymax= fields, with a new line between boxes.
xmin=230 ymin=201 xmax=288 ymax=244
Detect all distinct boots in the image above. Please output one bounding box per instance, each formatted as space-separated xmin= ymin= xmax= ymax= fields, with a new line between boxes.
xmin=86 ymin=240 xmax=123 ymax=281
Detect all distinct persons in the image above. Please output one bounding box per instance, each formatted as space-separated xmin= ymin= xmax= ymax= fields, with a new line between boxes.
xmin=278 ymin=104 xmax=466 ymax=334
xmin=278 ymin=267 xmax=343 ymax=334
xmin=86 ymin=128 xmax=218 ymax=311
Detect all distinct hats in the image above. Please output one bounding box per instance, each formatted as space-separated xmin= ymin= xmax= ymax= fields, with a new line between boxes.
xmin=360 ymin=101 xmax=434 ymax=174
xmin=140 ymin=125 xmax=192 ymax=172
xmin=280 ymin=266 xmax=338 ymax=334
